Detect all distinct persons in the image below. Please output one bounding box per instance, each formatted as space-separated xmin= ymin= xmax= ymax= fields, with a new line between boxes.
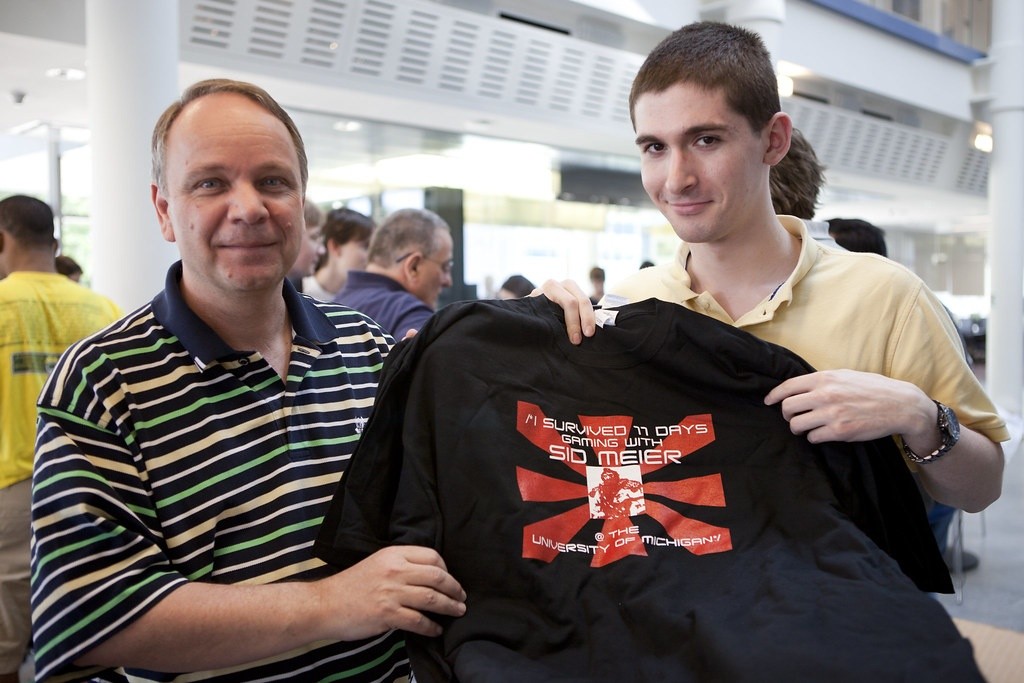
xmin=769 ymin=127 xmax=828 ymax=220
xmin=825 ymin=218 xmax=888 ymax=257
xmin=597 ymin=23 xmax=1008 ymax=514
xmin=0 ymin=80 xmax=655 ymax=683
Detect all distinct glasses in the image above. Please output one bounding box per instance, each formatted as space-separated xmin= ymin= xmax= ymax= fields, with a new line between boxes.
xmin=396 ymin=252 xmax=454 ymax=273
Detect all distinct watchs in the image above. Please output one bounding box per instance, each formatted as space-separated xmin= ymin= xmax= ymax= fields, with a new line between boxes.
xmin=901 ymin=399 xmax=960 ymax=464
